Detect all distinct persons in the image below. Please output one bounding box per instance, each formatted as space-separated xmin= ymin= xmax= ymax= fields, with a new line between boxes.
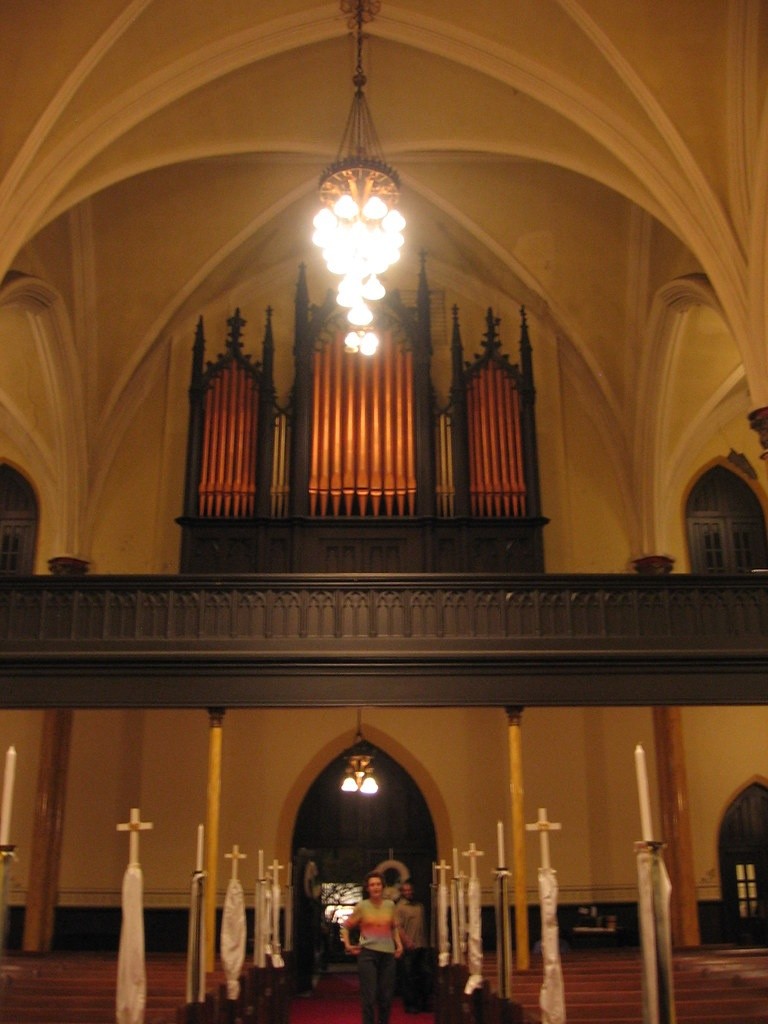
xmin=341 ymin=870 xmax=403 ymax=1024
xmin=396 ymin=881 xmax=426 ymax=1012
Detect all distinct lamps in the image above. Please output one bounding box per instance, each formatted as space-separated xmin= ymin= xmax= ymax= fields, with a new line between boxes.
xmin=309 ymin=0 xmax=405 ymax=353
xmin=341 ymin=706 xmax=380 ymax=793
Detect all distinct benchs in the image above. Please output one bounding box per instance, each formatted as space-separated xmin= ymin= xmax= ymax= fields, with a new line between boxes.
xmin=0 ymin=949 xmax=295 ymax=1024
xmin=445 ymin=945 xmax=768 ymax=1024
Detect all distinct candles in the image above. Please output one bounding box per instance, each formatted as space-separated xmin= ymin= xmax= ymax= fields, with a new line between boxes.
xmin=259 ymin=849 xmax=264 ymax=878
xmin=197 ymin=823 xmax=204 ymax=871
xmin=453 ymin=847 xmax=459 ymax=878
xmin=0 ymin=746 xmax=17 ymax=847
xmin=497 ymin=821 xmax=505 ymax=868
xmin=634 ymin=742 xmax=654 ymax=841
